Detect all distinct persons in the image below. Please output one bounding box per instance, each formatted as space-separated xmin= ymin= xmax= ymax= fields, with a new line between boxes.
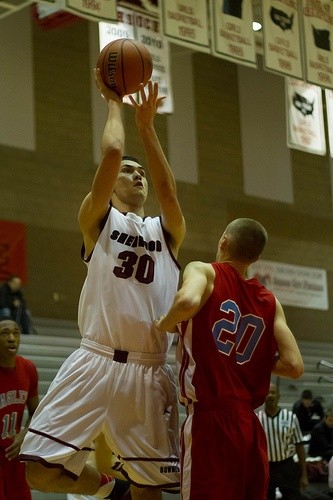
xmin=155 ymin=217 xmax=305 ymax=500
xmin=0 ymin=275 xmax=33 ymax=335
xmin=254 ymin=383 xmax=309 ymax=500
xmin=0 ymin=317 xmax=40 ymax=500
xmin=17 ymin=68 xmax=187 ymax=500
xmin=292 ymin=390 xmax=326 ymax=436
xmin=307 ymin=407 xmax=333 ymax=495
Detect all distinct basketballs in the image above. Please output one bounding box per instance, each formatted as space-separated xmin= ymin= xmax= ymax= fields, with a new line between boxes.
xmin=96 ymin=38 xmax=153 ymax=95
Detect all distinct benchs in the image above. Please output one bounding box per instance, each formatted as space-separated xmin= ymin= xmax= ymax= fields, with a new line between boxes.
xmin=14 ymin=315 xmax=333 ymax=464
